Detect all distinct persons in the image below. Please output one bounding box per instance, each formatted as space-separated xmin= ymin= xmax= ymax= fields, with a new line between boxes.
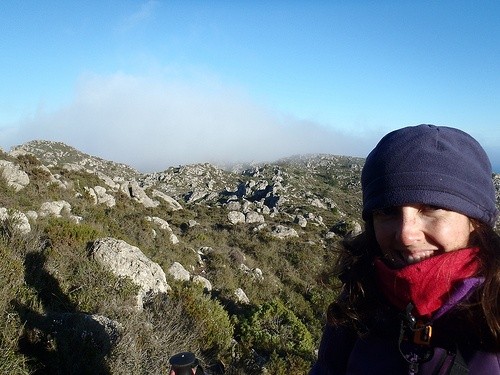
xmin=306 ymin=124 xmax=500 ymax=375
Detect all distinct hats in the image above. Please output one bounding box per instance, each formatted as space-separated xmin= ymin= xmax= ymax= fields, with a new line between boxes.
xmin=361 ymin=123 xmax=497 ymax=226
